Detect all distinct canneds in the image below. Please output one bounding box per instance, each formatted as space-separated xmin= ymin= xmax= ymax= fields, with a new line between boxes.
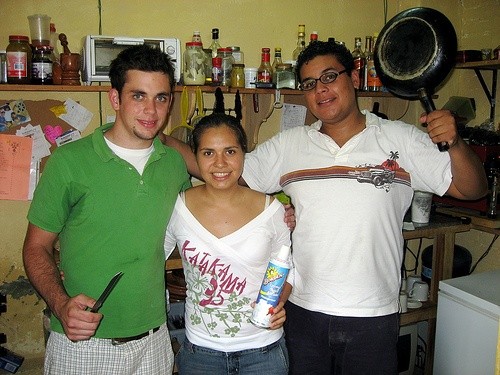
xmin=183 ymin=42 xmax=245 ymax=87
xmin=0 ymin=35 xmax=57 ymax=85
xmin=273 ymin=63 xmax=296 ymax=91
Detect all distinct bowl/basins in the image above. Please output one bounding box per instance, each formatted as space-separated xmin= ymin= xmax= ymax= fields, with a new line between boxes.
xmin=165 ymin=271 xmax=187 ymax=297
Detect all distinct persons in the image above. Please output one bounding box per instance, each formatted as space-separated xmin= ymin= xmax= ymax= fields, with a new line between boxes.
xmin=21 ymin=44 xmax=296 ymax=375
xmin=59 ymin=114 xmax=292 ymax=375
xmin=156 ymin=38 xmax=489 ymax=375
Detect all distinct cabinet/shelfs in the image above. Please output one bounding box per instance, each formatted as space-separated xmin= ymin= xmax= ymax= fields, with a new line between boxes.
xmin=165 ymin=208 xmax=473 ymax=375
xmin=434 ymin=59 xmax=500 ymax=235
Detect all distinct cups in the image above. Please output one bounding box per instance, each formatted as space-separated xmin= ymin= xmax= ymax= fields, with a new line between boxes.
xmin=27 ymin=13 xmax=52 ymax=48
xmin=397 ymin=274 xmax=429 ymax=313
xmin=411 ymin=190 xmax=433 ymax=224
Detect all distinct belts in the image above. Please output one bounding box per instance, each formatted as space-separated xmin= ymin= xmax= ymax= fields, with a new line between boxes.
xmin=111 ymin=326 xmax=160 ymax=346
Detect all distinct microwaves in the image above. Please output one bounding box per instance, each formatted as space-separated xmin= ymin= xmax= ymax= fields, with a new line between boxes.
xmin=78 ymin=34 xmax=182 ymax=87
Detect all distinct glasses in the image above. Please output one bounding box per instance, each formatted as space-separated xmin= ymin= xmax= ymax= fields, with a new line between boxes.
xmin=298 ymin=67 xmax=350 ymax=91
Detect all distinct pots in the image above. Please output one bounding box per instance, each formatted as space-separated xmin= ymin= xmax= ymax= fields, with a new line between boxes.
xmin=372 ymin=7 xmax=457 ymax=152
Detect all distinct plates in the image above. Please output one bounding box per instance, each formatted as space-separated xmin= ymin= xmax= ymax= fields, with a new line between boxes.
xmin=407 ymin=301 xmax=423 ymax=309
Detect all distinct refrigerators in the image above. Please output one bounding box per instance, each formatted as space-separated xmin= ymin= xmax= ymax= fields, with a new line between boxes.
xmin=433 ymin=268 xmax=500 ymax=374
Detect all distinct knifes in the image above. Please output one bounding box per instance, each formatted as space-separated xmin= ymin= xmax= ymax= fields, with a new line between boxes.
xmin=70 ymin=271 xmax=122 ymax=343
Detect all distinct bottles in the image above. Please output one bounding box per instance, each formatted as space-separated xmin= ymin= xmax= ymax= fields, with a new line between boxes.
xmin=182 ymin=24 xmax=389 ymax=92
xmin=250 ymin=245 xmax=292 ymax=329
xmin=5 ymin=34 xmax=32 ymax=86
xmin=31 ymin=48 xmax=54 ymax=85
xmin=50 ymin=23 xmax=60 ymax=66
xmin=483 ymin=155 xmax=500 ymax=219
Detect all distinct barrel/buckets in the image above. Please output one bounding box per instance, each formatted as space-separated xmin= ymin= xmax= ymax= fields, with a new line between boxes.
xmin=421 ymin=243 xmax=472 ymax=290
